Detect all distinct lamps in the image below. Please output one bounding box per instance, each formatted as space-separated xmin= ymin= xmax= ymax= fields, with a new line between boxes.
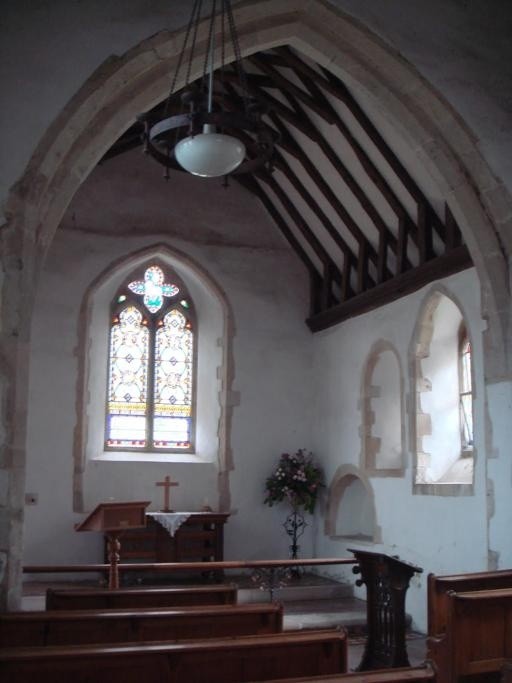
xmin=141 ymin=0 xmax=277 ymax=189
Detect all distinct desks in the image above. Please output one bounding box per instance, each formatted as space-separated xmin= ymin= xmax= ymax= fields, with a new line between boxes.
xmin=105 ymin=512 xmax=231 ymax=588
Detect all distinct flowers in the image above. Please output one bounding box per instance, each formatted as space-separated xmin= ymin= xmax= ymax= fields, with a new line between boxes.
xmin=264 ymin=446 xmax=325 ymax=519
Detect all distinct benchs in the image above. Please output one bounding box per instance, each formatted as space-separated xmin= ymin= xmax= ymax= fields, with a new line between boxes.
xmin=0 ymin=565 xmax=512 ymax=683
